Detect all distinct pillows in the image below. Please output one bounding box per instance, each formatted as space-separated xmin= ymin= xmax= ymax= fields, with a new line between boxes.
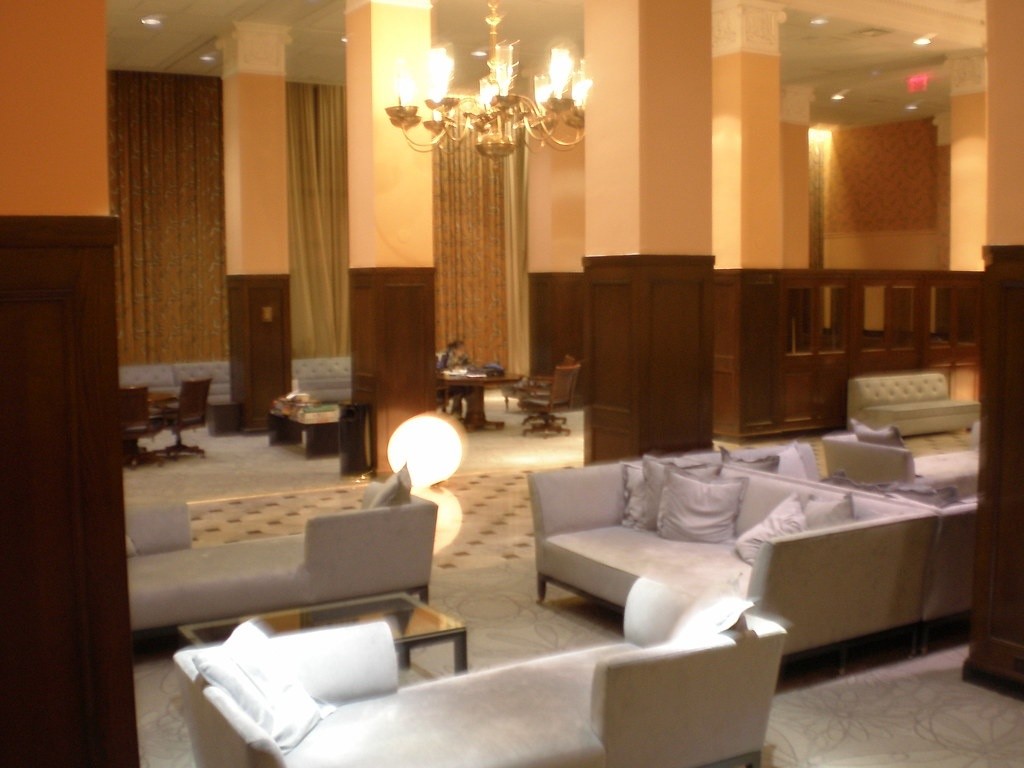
xmin=656 ymin=462 xmax=748 ymax=545
xmin=190 ymin=619 xmax=337 ymax=758
xmin=370 ymin=462 xmax=413 ymax=508
xmin=641 ymin=455 xmax=723 ymax=530
xmin=718 ymin=447 xmax=780 ymax=475
xmin=848 ymin=419 xmax=904 ymax=448
xmin=775 ymin=440 xmax=810 ymax=482
xmin=735 ymin=489 xmax=809 ymax=567
xmin=620 ymin=462 xmax=707 ymax=528
xmin=803 ymin=491 xmax=852 ymax=529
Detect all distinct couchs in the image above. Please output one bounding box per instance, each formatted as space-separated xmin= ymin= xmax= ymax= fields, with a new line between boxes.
xmin=125 ymin=462 xmax=438 ymax=657
xmin=174 ymin=569 xmax=788 ymax=768
xmin=528 ymin=419 xmax=980 ymax=657
xmin=847 ymin=373 xmax=980 ymax=437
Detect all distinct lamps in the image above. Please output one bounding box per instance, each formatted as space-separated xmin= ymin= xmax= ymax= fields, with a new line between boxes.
xmin=384 ymin=0 xmax=593 ymax=158
xmin=387 ymin=413 xmax=461 ymax=486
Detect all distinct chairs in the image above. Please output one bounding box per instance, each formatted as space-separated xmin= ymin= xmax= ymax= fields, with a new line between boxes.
xmin=120 ymin=384 xmax=155 ymax=469
xmin=151 ymin=375 xmax=214 ymax=465
xmin=503 ymin=354 xmax=577 ymax=416
xmin=518 ymin=364 xmax=581 ymax=437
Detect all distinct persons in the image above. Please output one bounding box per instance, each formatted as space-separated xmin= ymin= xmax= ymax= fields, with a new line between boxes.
xmin=436 ymin=340 xmax=516 ymax=421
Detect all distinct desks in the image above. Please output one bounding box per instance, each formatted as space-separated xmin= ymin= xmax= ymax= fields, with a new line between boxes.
xmin=122 ymin=389 xmax=177 ymax=459
xmin=436 ymin=368 xmax=522 ymax=432
xmin=268 ymin=407 xmax=341 ymax=459
xmin=178 ymin=592 xmax=468 ymax=677
